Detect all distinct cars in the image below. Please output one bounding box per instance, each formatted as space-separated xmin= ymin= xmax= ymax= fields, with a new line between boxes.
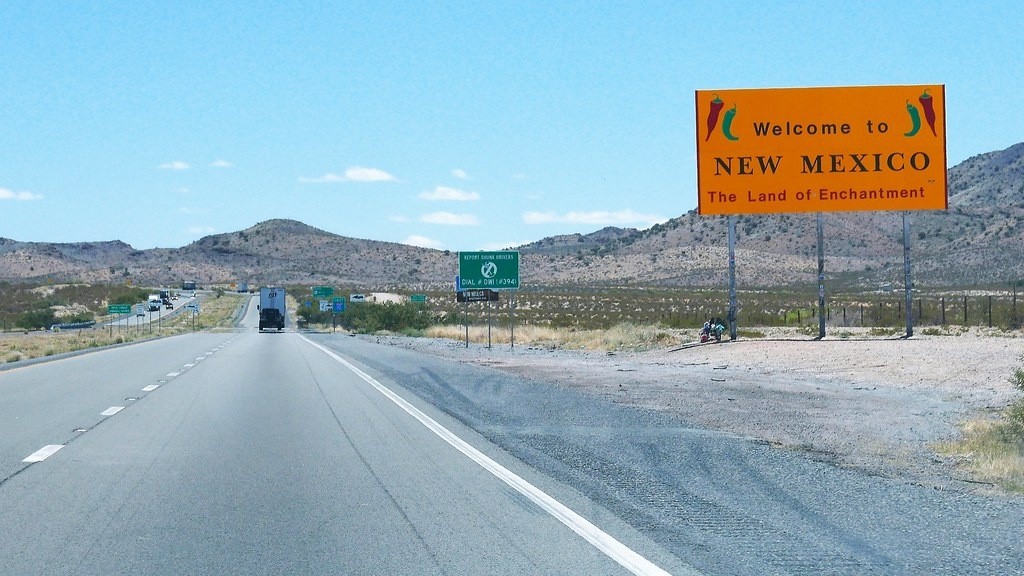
xmin=163 ymin=298 xmax=169 ymax=304
xmin=172 ymin=295 xmax=179 ymax=301
xmin=137 ymin=311 xmax=145 ymax=316
xmin=166 ymin=303 xmax=173 ymax=310
xmin=147 ymin=306 xmax=158 ymax=311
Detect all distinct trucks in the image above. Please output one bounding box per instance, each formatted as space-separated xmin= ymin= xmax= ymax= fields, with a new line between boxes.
xmin=159 ymin=289 xmax=171 ymax=299
xmin=257 ymin=287 xmax=286 ymax=330
